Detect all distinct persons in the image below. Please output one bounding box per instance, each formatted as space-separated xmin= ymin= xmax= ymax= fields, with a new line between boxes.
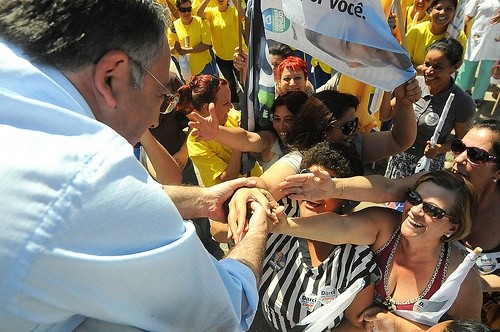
xmin=383 ymin=36 xmax=478 ymax=182
xmin=136 ymin=0 xmax=500 ymax=332
xmin=348 ymin=307 xmax=493 ymax=332
xmin=224 ymin=169 xmax=484 ymax=321
xmin=0 ymin=0 xmax=274 ymax=332
xmin=305 ymin=10 xmax=412 ymax=70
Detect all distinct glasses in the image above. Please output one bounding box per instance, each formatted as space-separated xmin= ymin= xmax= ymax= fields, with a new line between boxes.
xmin=330 ymin=117 xmax=360 ymax=135
xmin=94 ymin=52 xmax=180 ymax=114
xmin=178 ymin=7 xmax=192 ymax=12
xmin=405 ymin=187 xmax=455 ymax=221
xmin=450 ymin=138 xmax=500 ymax=166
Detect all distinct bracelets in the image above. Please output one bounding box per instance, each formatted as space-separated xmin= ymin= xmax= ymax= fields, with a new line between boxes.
xmin=337 ymin=178 xmax=347 ymax=200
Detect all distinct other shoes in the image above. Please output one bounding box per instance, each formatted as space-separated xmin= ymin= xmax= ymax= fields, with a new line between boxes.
xmin=232 ymin=102 xmax=240 ymax=111
